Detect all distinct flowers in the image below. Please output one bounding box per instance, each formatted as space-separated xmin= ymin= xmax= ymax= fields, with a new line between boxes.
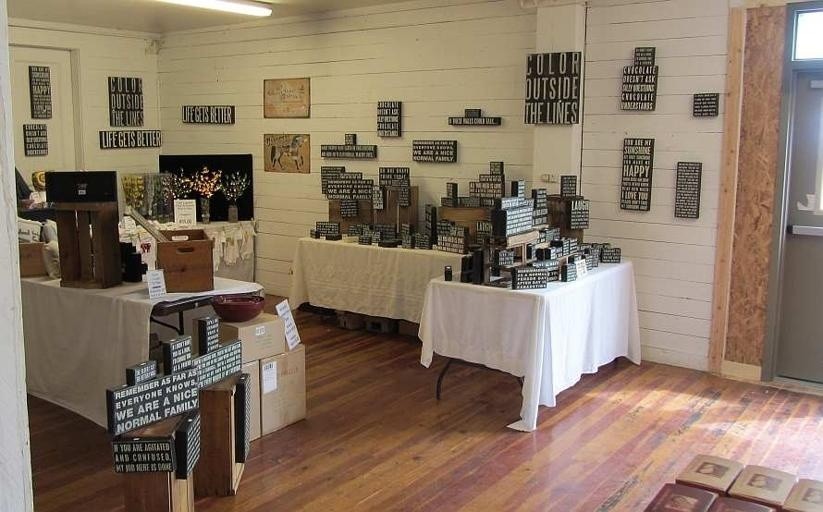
xmin=191 ymin=166 xmax=221 ymax=199
xmin=122 ymin=171 xmax=175 ymax=225
xmin=222 ymin=172 xmax=250 ymax=202
xmin=174 ymin=167 xmax=193 ymax=198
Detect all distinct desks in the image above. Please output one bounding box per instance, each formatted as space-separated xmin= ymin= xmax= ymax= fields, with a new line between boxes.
xmin=289 ymin=235 xmax=463 ymax=341
xmin=416 ymin=259 xmax=641 ymax=432
xmin=22 ymin=276 xmax=263 ymax=436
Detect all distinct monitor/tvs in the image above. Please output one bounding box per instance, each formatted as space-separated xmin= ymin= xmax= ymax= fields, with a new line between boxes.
xmin=45 ymin=171 xmax=117 ymax=202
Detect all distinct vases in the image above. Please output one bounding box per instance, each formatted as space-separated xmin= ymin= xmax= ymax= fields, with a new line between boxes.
xmin=200 ymin=197 xmax=211 ymax=224
xmin=228 ymin=205 xmax=238 ymax=223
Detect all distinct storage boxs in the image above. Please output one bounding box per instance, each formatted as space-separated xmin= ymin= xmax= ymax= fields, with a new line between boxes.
xmin=19 ymin=241 xmax=47 ymax=277
xmin=241 ymin=362 xmax=260 ymax=443
xmin=193 ymin=312 xmax=285 ymax=362
xmin=260 ymin=342 xmax=307 ymax=437
xmin=156 ymin=228 xmax=215 ymax=293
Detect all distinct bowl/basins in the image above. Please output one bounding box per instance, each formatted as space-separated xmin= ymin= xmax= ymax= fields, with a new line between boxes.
xmin=209 ymin=293 xmax=265 ymax=323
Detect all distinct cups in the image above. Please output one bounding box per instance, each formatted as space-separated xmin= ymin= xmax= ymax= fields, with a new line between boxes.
xmin=444 ymin=265 xmax=452 ymax=281
